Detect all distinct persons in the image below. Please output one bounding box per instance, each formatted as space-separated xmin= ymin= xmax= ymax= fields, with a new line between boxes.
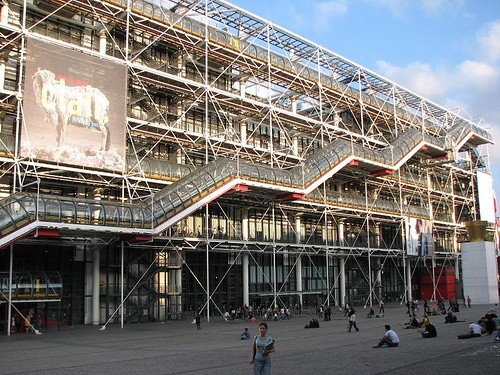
xmin=420 ymin=318 xmax=437 ymax=338
xmin=373 ymin=325 xmax=399 ymax=348
xmin=367 ymin=308 xmax=375 ymax=318
xmin=347 ymin=307 xmax=359 ymax=332
xmin=11 ymin=311 xmax=37 ymax=334
xmin=240 ymin=328 xmax=250 ymax=340
xmin=463 ymin=304 xmax=500 ymax=341
xmin=467 ymin=296 xmax=472 ymax=308
xmin=222 ymin=303 xmax=350 ymax=329
xmin=194 ymin=312 xmax=202 ymax=331
xmin=376 ymin=295 xmax=460 ymax=328
xmin=249 ymin=323 xmax=275 ymax=375
xmin=462 ymin=296 xmax=466 ymax=309
xmin=415 ymin=219 xmax=432 ymax=255
